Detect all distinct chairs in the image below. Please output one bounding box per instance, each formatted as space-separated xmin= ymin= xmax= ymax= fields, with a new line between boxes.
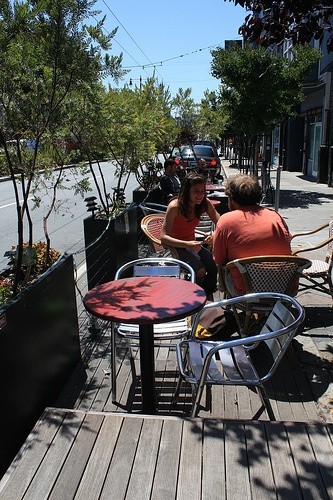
xmin=110 ymin=202 xmax=333 ymax=421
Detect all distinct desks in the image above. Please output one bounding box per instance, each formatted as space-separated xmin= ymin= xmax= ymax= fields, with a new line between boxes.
xmin=205 ymin=183 xmax=229 ymax=215
xmin=83 ymin=276 xmax=207 ymax=415
xmin=0 ymin=407 xmax=333 ymax=500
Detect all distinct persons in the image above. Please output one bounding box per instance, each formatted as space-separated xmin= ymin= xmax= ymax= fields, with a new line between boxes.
xmin=160 ymin=173 xmax=221 ymax=299
xmin=212 ymin=175 xmax=292 ymax=295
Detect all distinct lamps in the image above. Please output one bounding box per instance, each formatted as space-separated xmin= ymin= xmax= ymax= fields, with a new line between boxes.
xmin=117 ymin=189 xmax=126 ymax=204
xmin=3 ymin=251 xmax=16 ymax=270
xmin=83 ymin=197 xmax=99 ymax=218
xmin=141 ymin=175 xmax=148 ymax=188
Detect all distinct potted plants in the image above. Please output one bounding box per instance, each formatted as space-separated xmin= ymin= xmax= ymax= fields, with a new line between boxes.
xmin=0 ymin=0 xmax=164 ymax=441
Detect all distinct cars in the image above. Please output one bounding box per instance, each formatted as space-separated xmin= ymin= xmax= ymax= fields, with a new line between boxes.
xmin=167 ymin=144 xmax=222 ymax=178
xmin=195 ymin=140 xmax=218 ymax=156
